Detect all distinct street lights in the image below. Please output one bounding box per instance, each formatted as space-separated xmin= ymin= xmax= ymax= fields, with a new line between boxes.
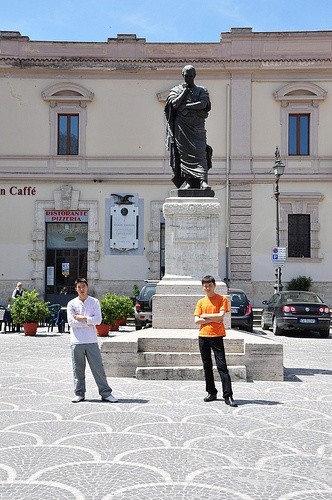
xmin=274 ymin=146 xmax=288 ymax=293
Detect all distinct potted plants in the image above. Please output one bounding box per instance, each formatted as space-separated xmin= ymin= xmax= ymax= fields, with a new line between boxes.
xmin=8 ymin=288 xmax=52 ymax=335
xmin=96 ymin=292 xmax=135 ymax=337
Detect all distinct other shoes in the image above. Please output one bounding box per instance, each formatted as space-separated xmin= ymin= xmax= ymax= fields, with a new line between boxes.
xmin=203 ymin=390 xmax=217 ymax=402
xmin=225 ymin=395 xmax=238 ymax=407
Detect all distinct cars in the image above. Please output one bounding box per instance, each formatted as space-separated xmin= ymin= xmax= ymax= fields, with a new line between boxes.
xmin=227 ymin=289 xmax=254 ymax=332
xmin=261 ymin=291 xmax=331 ymax=337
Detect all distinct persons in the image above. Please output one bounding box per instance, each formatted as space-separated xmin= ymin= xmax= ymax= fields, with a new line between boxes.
xmin=12 ymin=282 xmax=26 ymax=332
xmin=164 ymin=64 xmax=214 ymax=189
xmin=67 ymin=278 xmax=120 ymax=403
xmin=193 ymin=274 xmax=238 ymax=407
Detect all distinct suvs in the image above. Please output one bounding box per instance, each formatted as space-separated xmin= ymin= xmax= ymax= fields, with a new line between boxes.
xmin=134 ymin=279 xmax=159 ymax=332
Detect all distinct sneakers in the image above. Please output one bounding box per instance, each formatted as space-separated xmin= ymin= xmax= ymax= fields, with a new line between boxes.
xmin=102 ymin=394 xmax=119 ymax=402
xmin=71 ymin=395 xmax=84 ymax=402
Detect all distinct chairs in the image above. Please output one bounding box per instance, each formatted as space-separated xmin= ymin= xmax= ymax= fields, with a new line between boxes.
xmin=232 ymin=296 xmax=239 ymax=301
xmin=0 ymin=305 xmax=14 ymax=334
